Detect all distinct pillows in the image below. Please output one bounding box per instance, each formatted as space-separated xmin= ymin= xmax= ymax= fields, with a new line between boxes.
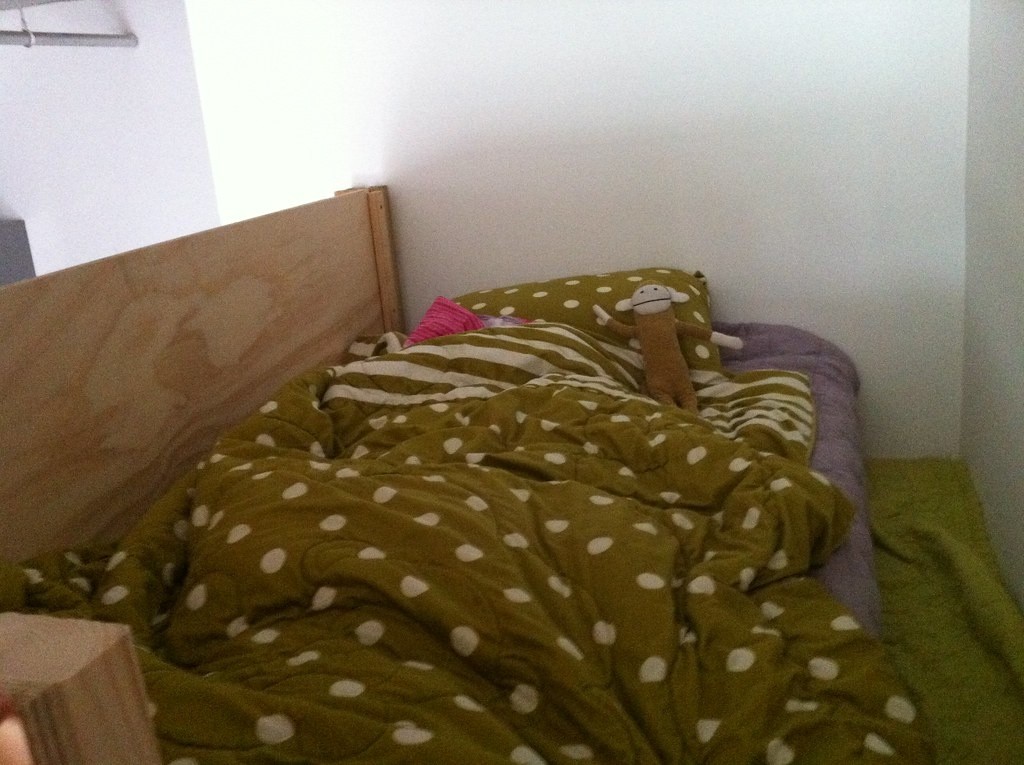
xmin=450 ymin=268 xmax=724 ymax=374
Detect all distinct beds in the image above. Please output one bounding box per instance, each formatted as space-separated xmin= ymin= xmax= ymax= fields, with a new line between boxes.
xmin=16 ymin=324 xmax=881 ymax=765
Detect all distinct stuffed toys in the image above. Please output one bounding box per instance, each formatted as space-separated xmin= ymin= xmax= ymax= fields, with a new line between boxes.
xmin=592 ymin=279 xmax=745 ymax=414
